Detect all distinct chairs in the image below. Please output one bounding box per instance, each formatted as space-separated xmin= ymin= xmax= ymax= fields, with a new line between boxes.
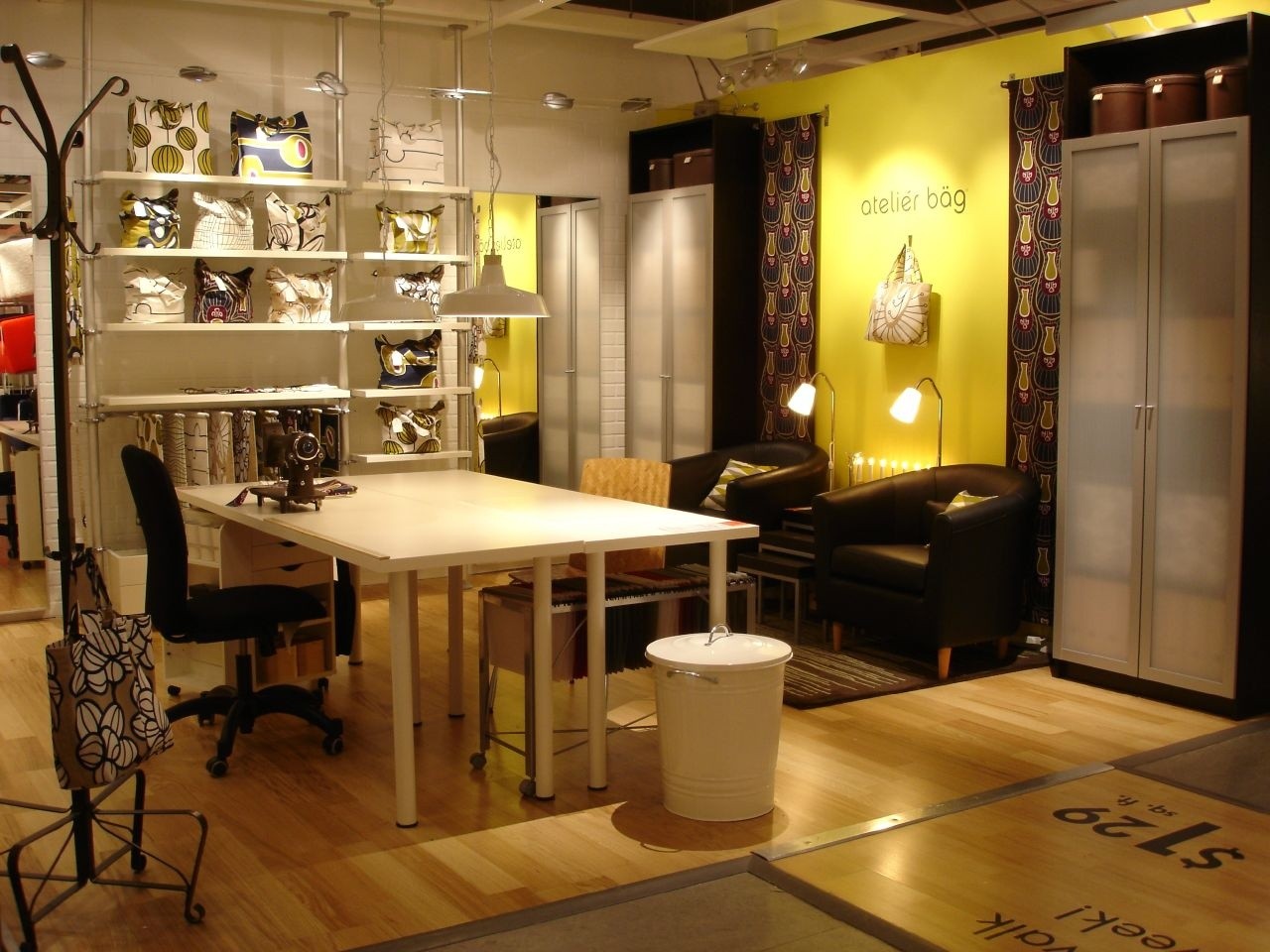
xmin=119 ymin=444 xmax=344 ymax=780
xmin=481 ymin=412 xmax=539 ymax=484
xmin=812 ymin=462 xmax=1039 ymax=681
xmin=666 ymin=441 xmax=829 ymax=574
xmin=487 ymin=458 xmax=672 ymax=714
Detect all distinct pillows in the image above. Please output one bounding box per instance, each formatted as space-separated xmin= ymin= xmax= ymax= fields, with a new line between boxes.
xmin=923 ymin=490 xmax=999 ymax=548
xmin=699 ymin=457 xmax=780 ymax=511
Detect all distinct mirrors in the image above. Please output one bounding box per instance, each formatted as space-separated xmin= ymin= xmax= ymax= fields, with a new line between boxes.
xmin=471 ymin=189 xmax=601 ymax=491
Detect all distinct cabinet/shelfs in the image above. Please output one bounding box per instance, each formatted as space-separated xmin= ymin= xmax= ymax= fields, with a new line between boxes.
xmin=77 ymin=0 xmax=478 ymax=697
xmin=1049 ymin=12 xmax=1270 ymax=722
xmin=626 ymin=111 xmax=764 ymax=464
xmin=536 ymin=200 xmax=600 ymax=490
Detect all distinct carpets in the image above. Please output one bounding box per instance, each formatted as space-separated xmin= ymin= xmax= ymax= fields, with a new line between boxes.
xmin=759 ymin=615 xmax=1049 ymax=708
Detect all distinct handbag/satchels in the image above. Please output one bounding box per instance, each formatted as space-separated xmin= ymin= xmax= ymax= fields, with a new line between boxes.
xmin=395 ymin=264 xmax=445 ymax=322
xmin=264 ymin=191 xmax=332 ymax=251
xmin=192 ymin=259 xmax=254 ymax=323
xmin=366 ymin=117 xmax=445 ymax=186
xmin=373 ymin=329 xmax=442 ymax=389
xmin=118 ymin=189 xmax=182 ymax=248
xmin=264 ymin=266 xmax=336 ymax=323
xmin=229 ymin=110 xmax=313 ymax=179
xmin=375 ymin=399 xmax=444 ymax=455
xmin=864 ymin=245 xmax=932 ymax=348
xmin=45 ymin=546 xmax=176 ymax=790
xmin=126 ymin=96 xmax=214 ymax=175
xmin=191 ymin=191 xmax=256 ymax=250
xmin=122 ymin=263 xmax=187 ymax=324
xmin=374 ymin=201 xmax=445 ymax=254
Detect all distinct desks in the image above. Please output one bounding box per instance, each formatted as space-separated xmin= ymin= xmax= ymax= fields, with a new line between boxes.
xmin=0 ymin=421 xmax=40 ymax=520
xmin=736 ymin=507 xmax=865 ymax=644
xmin=176 ymin=466 xmax=760 ymax=831
xmin=470 ymin=564 xmax=757 ymax=798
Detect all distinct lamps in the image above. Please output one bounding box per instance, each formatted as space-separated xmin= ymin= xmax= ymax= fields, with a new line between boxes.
xmin=435 ymin=0 xmax=552 ymax=318
xmin=787 ymin=373 xmax=943 ymax=492
xmin=715 ymin=29 xmax=808 ymax=95
xmin=474 ymin=358 xmax=502 ymax=416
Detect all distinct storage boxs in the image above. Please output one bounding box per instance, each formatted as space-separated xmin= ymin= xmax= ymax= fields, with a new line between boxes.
xmin=672 ymin=149 xmax=714 ymax=188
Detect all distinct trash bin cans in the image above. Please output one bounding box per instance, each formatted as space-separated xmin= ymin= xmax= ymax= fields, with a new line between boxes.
xmin=646 ymin=624 xmax=792 ymax=822
